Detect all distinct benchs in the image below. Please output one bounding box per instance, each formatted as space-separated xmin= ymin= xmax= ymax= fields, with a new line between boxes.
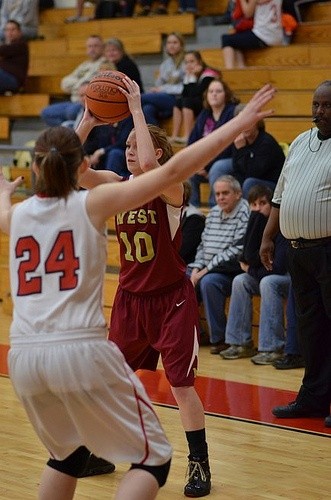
xmin=0 ymin=0 xmax=331 ymax=348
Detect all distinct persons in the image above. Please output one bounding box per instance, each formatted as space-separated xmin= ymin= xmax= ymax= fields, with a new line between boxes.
xmin=221 ymin=0 xmax=311 ymax=69
xmin=41 ymin=32 xmax=301 ymax=370
xmin=259 ymin=80 xmax=331 ymax=427
xmin=0 ymin=0 xmax=55 ymax=94
xmin=65 ymin=0 xmax=196 ymax=23
xmin=75 ymin=75 xmax=211 ymax=498
xmin=0 ymin=84 xmax=277 ymax=500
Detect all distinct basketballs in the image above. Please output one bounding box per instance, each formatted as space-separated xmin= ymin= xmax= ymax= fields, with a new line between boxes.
xmin=86 ymin=71 xmax=132 ymax=123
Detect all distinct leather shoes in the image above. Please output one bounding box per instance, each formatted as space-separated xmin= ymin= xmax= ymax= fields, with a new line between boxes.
xmin=272 ymin=401 xmax=330 ymax=418
xmin=324 ymin=417 xmax=331 ymax=428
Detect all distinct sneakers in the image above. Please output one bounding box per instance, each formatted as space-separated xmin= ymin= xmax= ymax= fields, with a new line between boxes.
xmin=273 ymin=354 xmax=307 ymax=370
xmin=82 ymin=454 xmax=116 ymax=477
xmin=184 ymin=455 xmax=211 ymax=498
xmin=250 ymin=352 xmax=283 ymax=365
xmin=219 ymin=345 xmax=257 ymax=360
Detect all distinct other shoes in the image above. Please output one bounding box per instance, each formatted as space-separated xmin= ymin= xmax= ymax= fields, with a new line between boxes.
xmin=210 ymin=343 xmax=229 ymax=354
xmin=169 ymin=136 xmax=188 ymax=145
